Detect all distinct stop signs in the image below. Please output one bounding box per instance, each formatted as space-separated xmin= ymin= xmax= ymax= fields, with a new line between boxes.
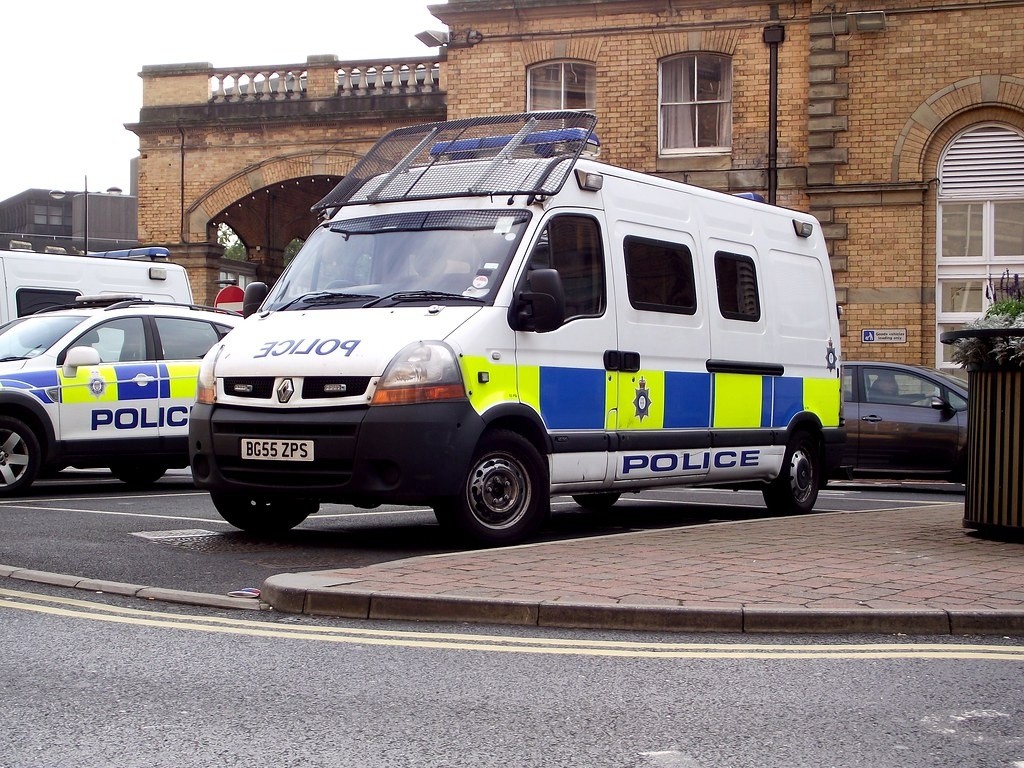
xmin=213 ymin=285 xmax=245 ymax=317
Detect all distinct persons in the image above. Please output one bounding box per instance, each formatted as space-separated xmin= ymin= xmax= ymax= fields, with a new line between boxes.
xmin=867 ymin=371 xmax=937 ymax=405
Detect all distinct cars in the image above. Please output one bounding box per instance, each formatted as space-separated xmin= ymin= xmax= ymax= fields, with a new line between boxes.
xmin=818 ymin=359 xmax=969 ymax=493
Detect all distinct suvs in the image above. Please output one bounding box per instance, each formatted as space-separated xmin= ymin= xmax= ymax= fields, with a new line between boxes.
xmin=0 ymin=296 xmax=249 ymax=497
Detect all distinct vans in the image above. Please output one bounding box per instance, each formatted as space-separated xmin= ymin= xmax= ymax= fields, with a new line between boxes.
xmin=0 ymin=242 xmax=194 ymax=327
xmin=188 ymin=108 xmax=846 ymax=548
